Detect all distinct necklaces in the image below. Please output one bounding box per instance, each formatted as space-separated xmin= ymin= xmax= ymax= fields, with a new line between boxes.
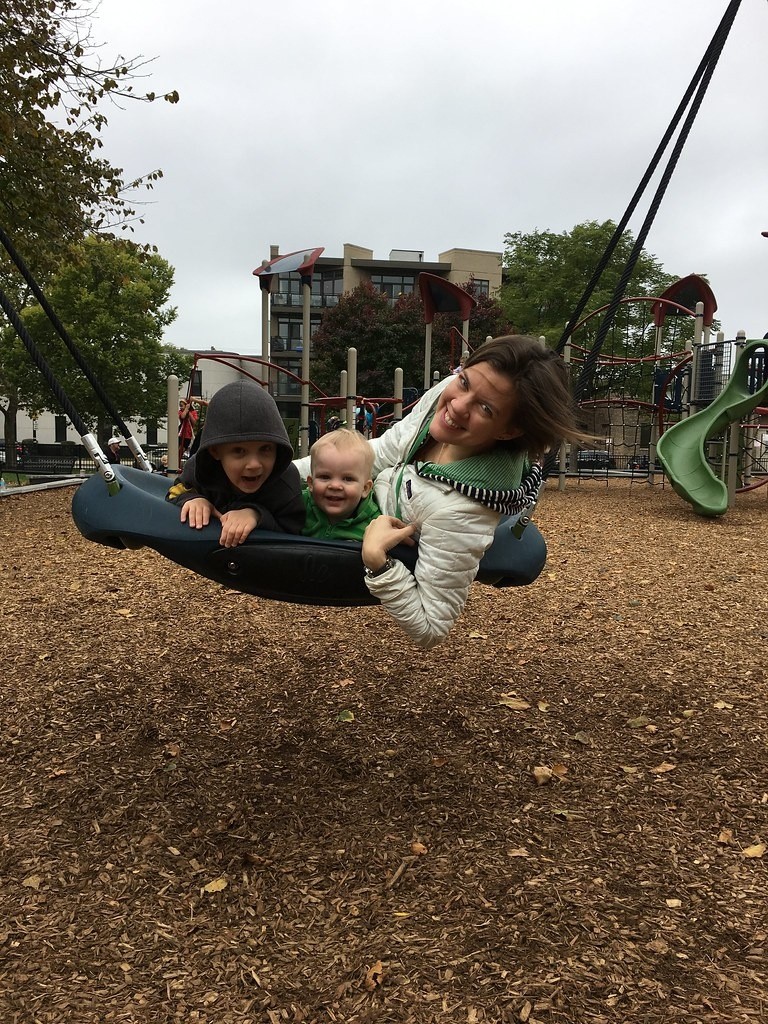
xmin=437 ymin=443 xmax=445 ymax=464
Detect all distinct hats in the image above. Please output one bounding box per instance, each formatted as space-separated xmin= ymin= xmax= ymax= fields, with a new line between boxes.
xmin=108 ymin=438 xmax=121 ymax=446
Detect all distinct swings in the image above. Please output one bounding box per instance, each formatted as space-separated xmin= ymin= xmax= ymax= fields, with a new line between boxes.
xmin=0 ymin=0 xmax=744 ymax=608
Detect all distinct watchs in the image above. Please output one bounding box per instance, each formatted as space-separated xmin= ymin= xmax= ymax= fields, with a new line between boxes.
xmin=363 ymin=557 xmax=394 ymax=579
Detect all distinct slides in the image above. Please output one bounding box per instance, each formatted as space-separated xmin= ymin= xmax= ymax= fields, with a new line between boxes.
xmin=656 ymin=340 xmax=768 ymax=515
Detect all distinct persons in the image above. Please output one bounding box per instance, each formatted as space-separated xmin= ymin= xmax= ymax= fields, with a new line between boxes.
xmin=103 ymin=437 xmax=122 ymax=465
xmin=326 ymin=402 xmax=379 ymax=432
xmin=154 ymin=454 xmax=168 ymax=477
xmin=453 ymin=356 xmax=468 ymax=374
xmin=164 ymin=378 xmax=306 ymax=548
xmin=301 ymin=426 xmax=381 ymax=543
xmin=178 ymin=398 xmax=206 ymax=463
xmin=291 ymin=335 xmax=573 ymax=648
